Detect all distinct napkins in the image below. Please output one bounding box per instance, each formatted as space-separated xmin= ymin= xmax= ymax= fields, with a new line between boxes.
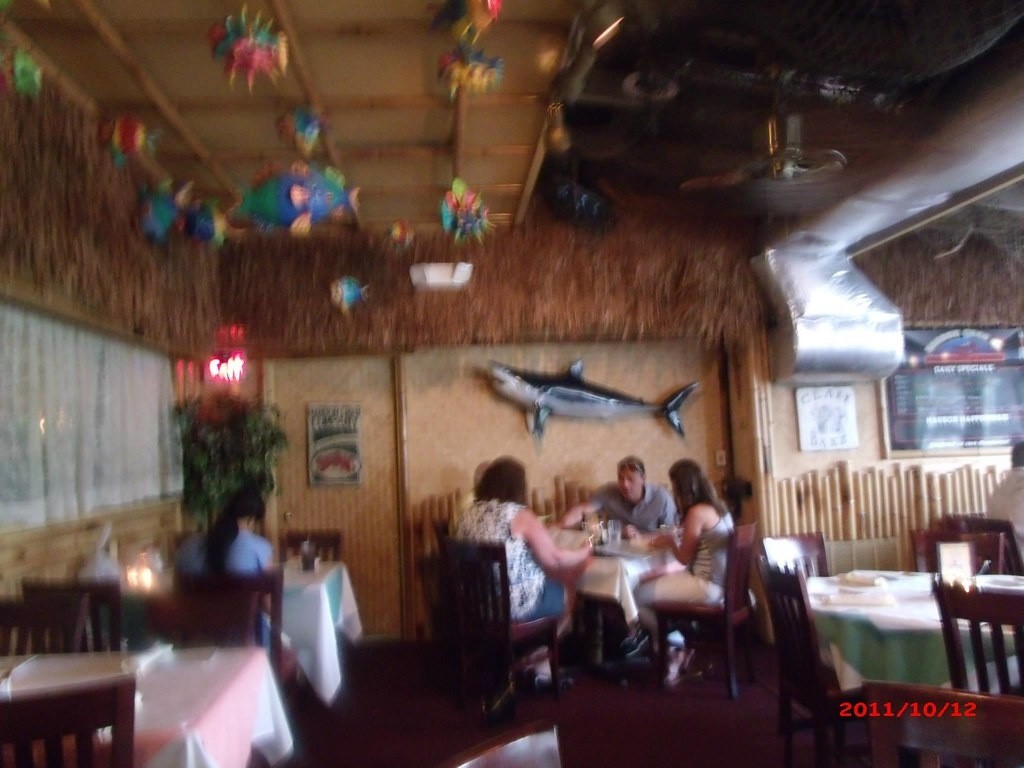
xmin=826 ymin=592 xmax=893 ymax=604
xmin=120 ymin=640 xmax=174 ymax=673
xmin=837 ymin=572 xmax=885 ymax=585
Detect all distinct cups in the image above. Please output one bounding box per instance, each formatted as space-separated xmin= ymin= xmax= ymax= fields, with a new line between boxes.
xmin=607 ymin=520 xmax=621 ymax=546
xmin=301 ymin=541 xmax=315 ymax=572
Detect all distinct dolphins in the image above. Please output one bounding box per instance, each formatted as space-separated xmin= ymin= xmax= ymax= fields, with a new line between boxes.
xmin=484 ymin=354 xmax=705 ymax=441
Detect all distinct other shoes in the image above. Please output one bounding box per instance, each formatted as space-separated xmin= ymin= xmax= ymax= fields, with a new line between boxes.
xmin=623 ymin=629 xmax=649 ymax=656
xmin=665 ymin=646 xmax=693 ymax=685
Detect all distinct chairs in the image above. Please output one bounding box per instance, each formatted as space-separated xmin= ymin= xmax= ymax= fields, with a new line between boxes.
xmin=0 ymin=514 xmax=1024 ymax=768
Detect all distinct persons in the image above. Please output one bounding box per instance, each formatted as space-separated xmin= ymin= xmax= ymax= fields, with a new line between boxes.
xmin=634 ymin=460 xmax=735 ymax=682
xmin=988 ymin=442 xmax=1024 ymax=568
xmin=447 ymin=456 xmax=592 ymax=690
xmin=175 ymin=493 xmax=300 ymax=697
xmin=547 ymin=456 xmax=679 ymax=657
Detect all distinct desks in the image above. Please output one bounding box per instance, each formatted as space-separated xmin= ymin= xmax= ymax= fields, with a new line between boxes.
xmin=550 ymin=529 xmax=689 ymax=689
xmin=0 ymin=648 xmax=294 ymax=768
xmin=805 ymin=570 xmax=1024 ymax=695
xmin=85 ymin=560 xmax=361 ymax=704
